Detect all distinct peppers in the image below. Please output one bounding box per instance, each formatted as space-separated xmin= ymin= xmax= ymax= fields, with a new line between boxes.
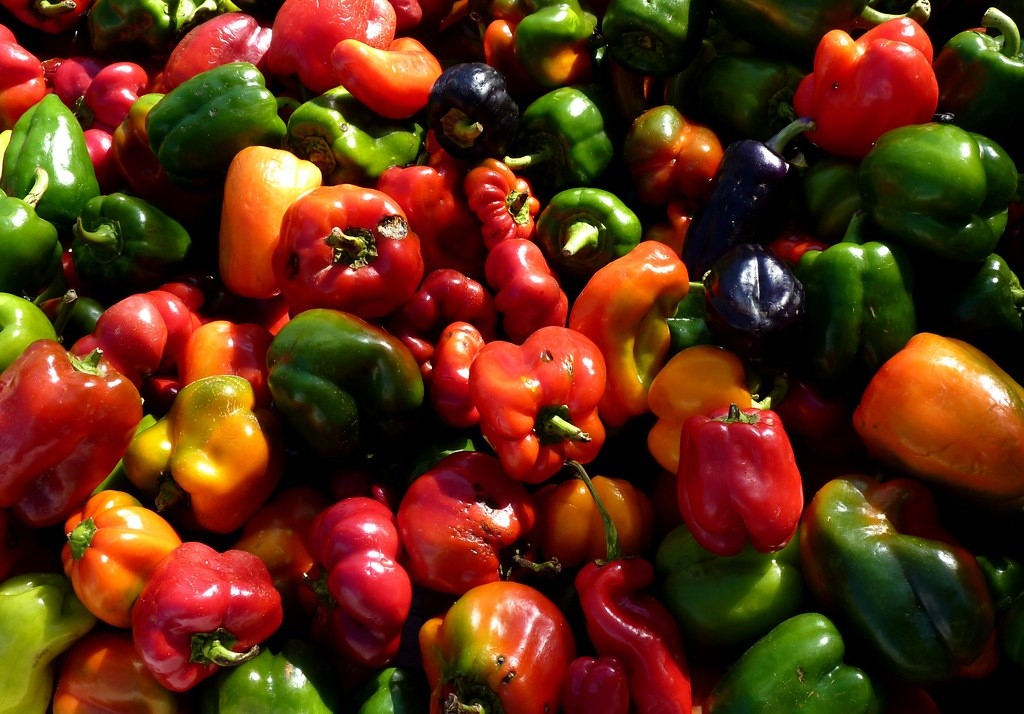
xmin=0 ymin=0 xmax=1024 ymax=714
